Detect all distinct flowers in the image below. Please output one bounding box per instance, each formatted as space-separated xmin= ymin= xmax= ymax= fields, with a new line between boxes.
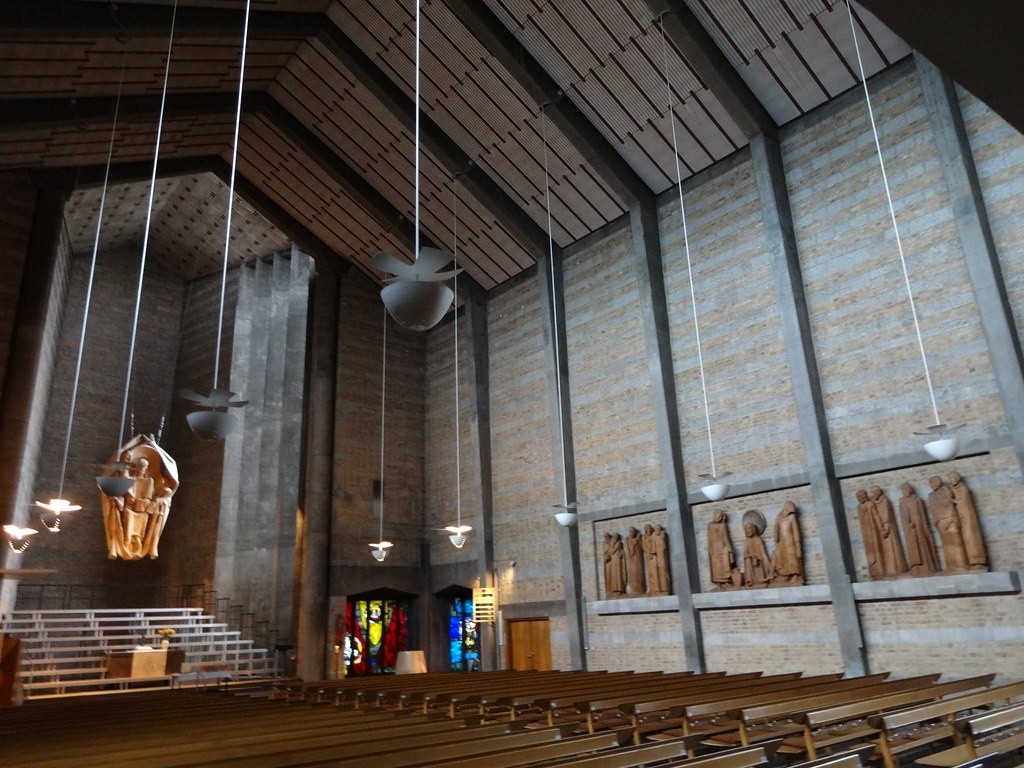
xmin=158 ymin=628 xmax=175 ymax=641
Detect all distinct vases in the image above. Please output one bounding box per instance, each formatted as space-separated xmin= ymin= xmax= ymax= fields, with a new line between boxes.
xmin=162 ymin=639 xmax=169 ymax=650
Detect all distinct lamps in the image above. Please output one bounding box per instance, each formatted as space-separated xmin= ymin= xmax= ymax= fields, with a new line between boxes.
xmin=659 ymin=13 xmax=734 ymax=501
xmin=445 ymin=174 xmax=472 ymax=548
xmin=181 ymin=0 xmax=251 ymax=441
xmin=92 ymin=1 xmax=179 ymax=496
xmin=35 ymin=37 xmax=131 ymax=534
xmin=369 ymin=230 xmax=394 ymax=562
xmin=2 ymin=524 xmax=40 ymax=553
xmin=845 ymin=0 xmax=967 ymax=461
xmin=542 ymin=105 xmax=581 ymax=526
xmin=371 ymin=0 xmax=464 ymax=331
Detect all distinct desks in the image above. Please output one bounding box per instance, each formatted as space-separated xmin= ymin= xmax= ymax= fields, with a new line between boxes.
xmin=105 ymin=649 xmax=185 ymax=690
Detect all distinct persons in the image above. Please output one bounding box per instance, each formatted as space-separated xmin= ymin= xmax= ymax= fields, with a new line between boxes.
xmin=707 ymin=500 xmax=805 ymax=591
xmin=602 ymin=524 xmax=672 ymax=597
xmin=112 ymin=450 xmax=177 ymax=559
xmin=855 ymin=471 xmax=990 ymax=582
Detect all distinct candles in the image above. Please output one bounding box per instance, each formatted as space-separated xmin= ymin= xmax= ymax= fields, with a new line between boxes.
xmin=335 ymin=646 xmax=340 ymax=654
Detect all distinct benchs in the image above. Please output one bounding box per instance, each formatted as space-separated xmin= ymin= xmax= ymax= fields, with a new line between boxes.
xmin=0 ymin=668 xmax=1024 ymax=768
xmin=0 ymin=607 xmax=299 ymax=700
